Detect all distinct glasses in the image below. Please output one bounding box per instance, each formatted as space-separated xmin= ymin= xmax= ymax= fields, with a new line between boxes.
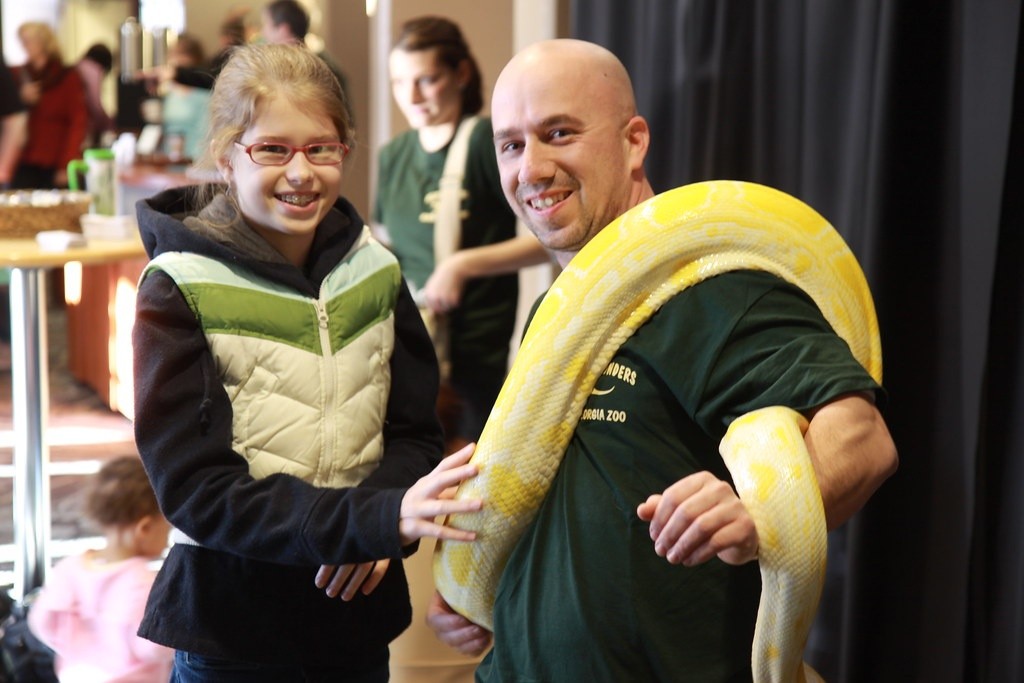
xmin=233 ymin=141 xmax=349 ymax=165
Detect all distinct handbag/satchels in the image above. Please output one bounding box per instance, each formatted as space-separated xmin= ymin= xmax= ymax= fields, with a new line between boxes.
xmin=0 ymin=620 xmax=61 ymax=683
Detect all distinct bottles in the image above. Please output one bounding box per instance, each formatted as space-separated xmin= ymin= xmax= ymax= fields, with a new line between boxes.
xmin=120 ymin=16 xmax=169 ymax=70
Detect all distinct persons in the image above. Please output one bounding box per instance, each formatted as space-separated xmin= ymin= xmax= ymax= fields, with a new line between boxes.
xmin=265 ymin=0 xmax=353 ymax=128
xmin=136 ymin=42 xmax=484 ymax=683
xmin=27 ymin=455 xmax=178 ymax=683
xmin=425 ymin=38 xmax=900 ymax=683
xmin=370 ymin=16 xmax=551 ymax=441
xmin=157 ymin=14 xmax=258 ymax=161
xmin=0 ymin=22 xmax=115 ymax=189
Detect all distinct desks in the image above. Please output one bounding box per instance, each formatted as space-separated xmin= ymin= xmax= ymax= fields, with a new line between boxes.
xmin=0 ymin=213 xmax=147 ymax=620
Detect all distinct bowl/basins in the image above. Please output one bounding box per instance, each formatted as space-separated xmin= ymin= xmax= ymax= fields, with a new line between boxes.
xmin=80 ymin=215 xmax=135 ymax=251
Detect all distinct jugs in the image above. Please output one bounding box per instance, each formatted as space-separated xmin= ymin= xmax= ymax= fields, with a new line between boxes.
xmin=68 ymin=147 xmax=118 ymax=216
